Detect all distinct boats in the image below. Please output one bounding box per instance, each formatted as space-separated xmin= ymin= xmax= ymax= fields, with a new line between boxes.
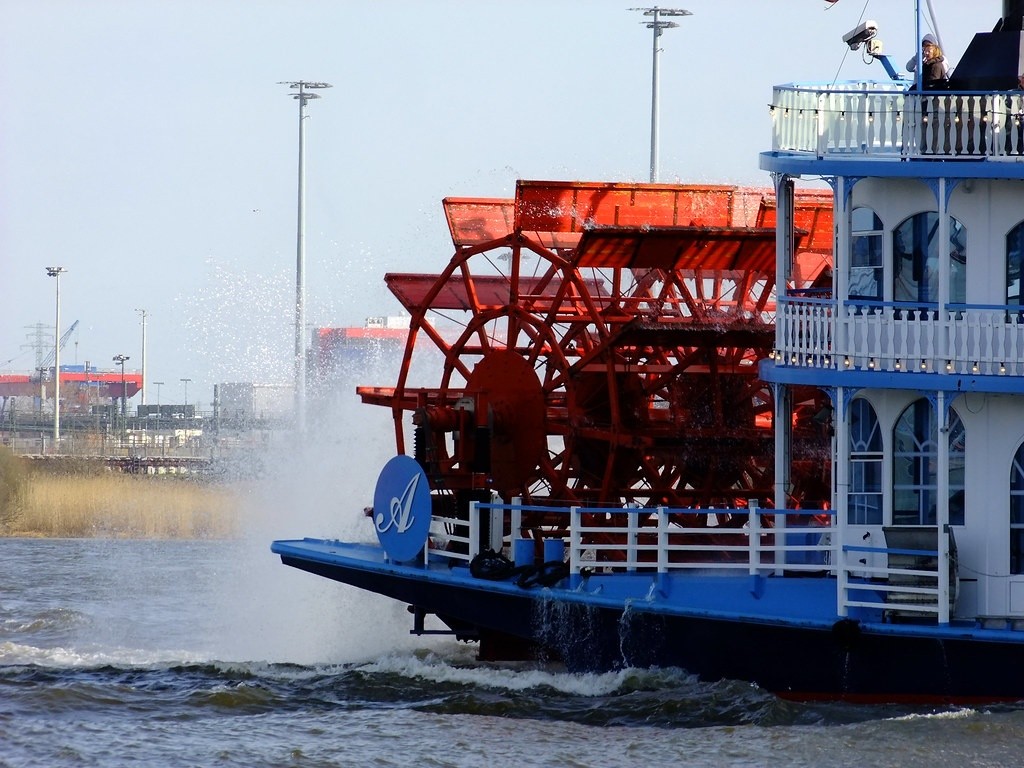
xmin=268 ymin=0 xmax=1023 ymax=708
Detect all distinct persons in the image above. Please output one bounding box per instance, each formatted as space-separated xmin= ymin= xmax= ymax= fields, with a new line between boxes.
xmin=906 ymin=33 xmax=949 ymax=152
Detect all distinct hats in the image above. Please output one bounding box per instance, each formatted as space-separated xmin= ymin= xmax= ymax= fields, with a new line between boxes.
xmin=922 ymin=34 xmax=936 ymax=45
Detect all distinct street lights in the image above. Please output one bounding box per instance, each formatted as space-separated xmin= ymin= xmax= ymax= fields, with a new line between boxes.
xmin=626 ymin=5 xmax=694 ymax=184
xmin=275 ymin=76 xmax=336 ymax=440
xmin=136 ymin=307 xmax=148 ymax=405
xmin=112 ymin=355 xmax=131 ymax=413
xmin=180 ymin=379 xmax=192 ymax=449
xmin=152 ymin=381 xmax=166 ymax=444
xmin=46 ymin=266 xmax=70 ymax=455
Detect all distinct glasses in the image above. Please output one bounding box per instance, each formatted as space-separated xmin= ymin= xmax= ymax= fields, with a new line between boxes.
xmin=924 ymin=50 xmax=933 ymax=54
